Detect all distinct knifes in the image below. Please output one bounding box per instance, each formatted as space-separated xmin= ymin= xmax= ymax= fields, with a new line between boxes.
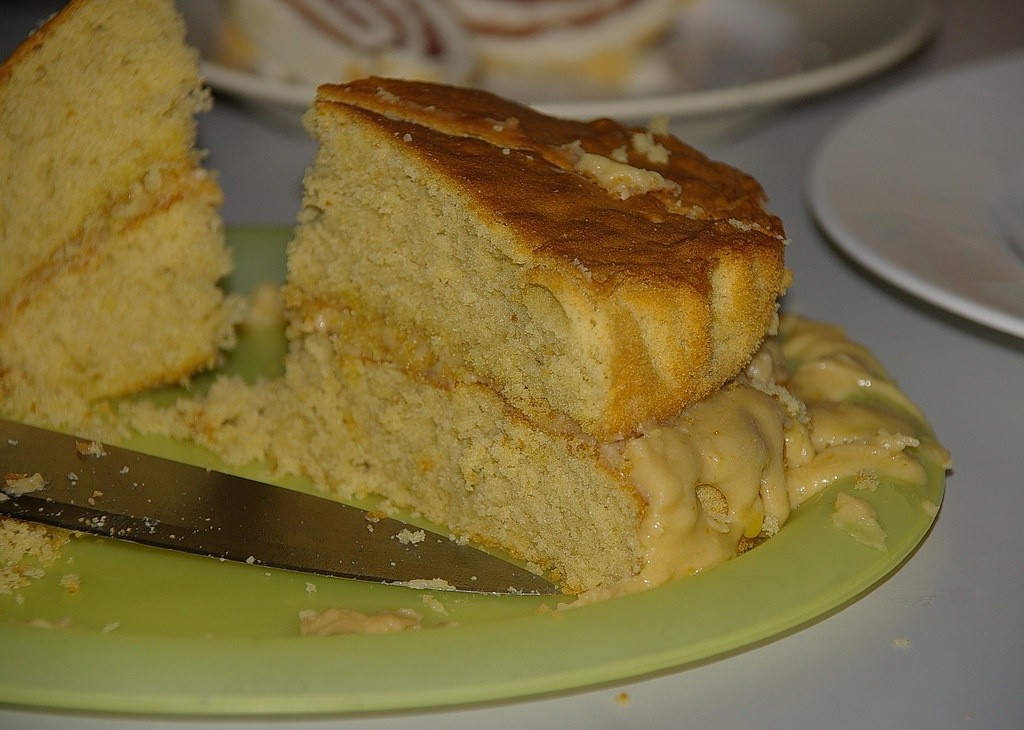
xmin=1 ymin=419 xmax=564 ymax=596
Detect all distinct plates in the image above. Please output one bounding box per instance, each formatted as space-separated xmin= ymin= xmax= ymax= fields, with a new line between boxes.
xmin=0 ymin=215 xmax=945 ymax=719
xmin=804 ymin=52 xmax=1024 ymax=340
xmin=182 ymin=1 xmax=946 ymax=142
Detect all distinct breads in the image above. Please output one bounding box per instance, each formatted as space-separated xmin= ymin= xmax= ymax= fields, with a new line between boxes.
xmin=276 ymin=75 xmax=808 ymax=591
xmin=0 ymin=1 xmax=245 ymax=430
